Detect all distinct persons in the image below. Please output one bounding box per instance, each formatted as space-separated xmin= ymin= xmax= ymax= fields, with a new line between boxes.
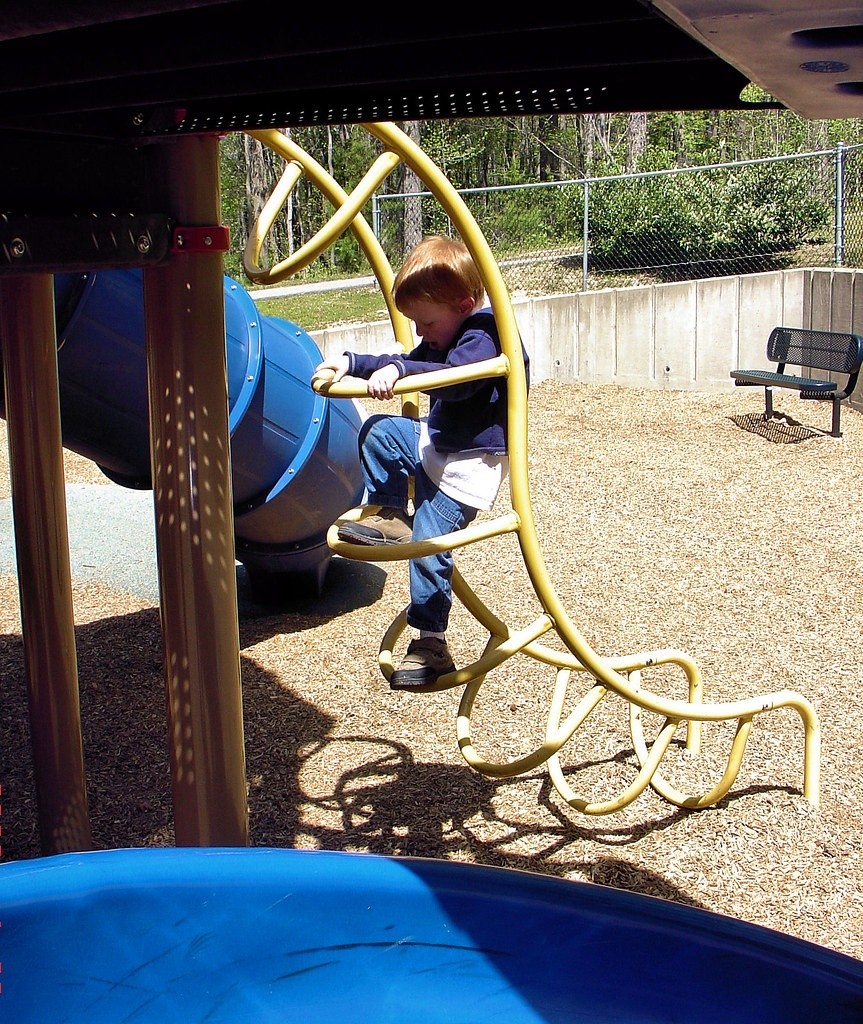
xmin=314 ymin=235 xmax=530 ymax=688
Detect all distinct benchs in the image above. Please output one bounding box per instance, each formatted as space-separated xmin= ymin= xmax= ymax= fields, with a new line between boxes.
xmin=730 ymin=326 xmax=863 ymax=437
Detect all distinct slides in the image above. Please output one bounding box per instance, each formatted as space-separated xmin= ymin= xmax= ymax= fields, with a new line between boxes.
xmin=51 ymin=263 xmax=367 ymax=578
xmin=0 ymin=845 xmax=862 ymax=1023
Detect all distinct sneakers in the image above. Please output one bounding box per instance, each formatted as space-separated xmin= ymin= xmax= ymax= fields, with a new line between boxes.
xmin=338 ymin=508 xmax=413 ymax=546
xmin=390 ymin=637 xmax=457 ymax=690
